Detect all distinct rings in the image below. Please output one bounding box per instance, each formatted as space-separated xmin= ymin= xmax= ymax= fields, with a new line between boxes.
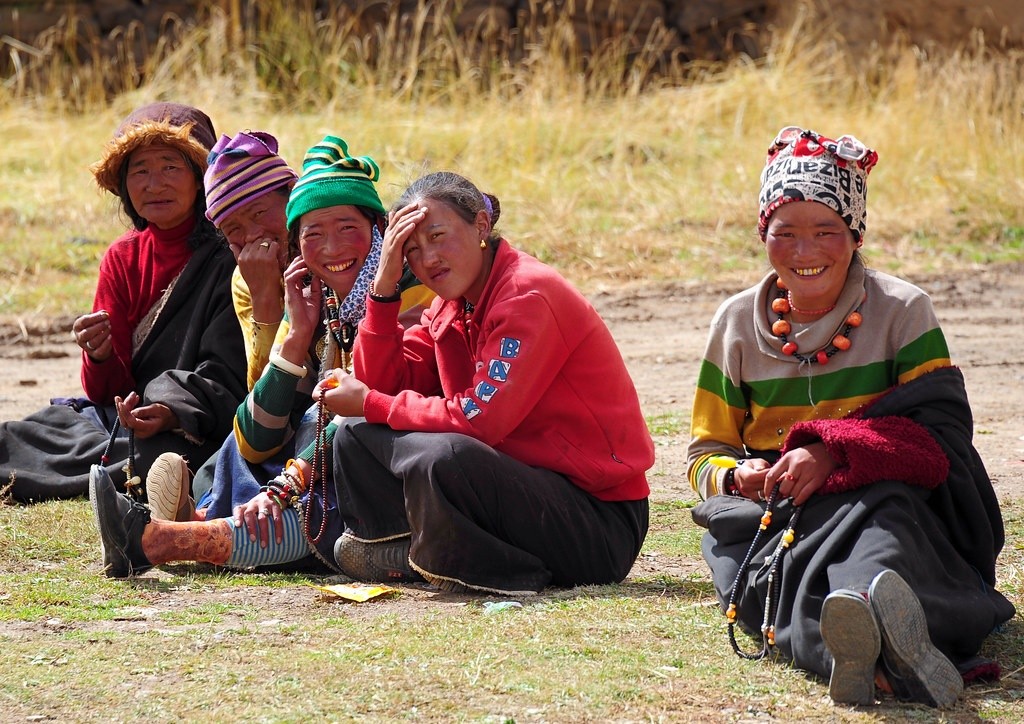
xmin=785 ymin=473 xmax=797 ymax=481
xmin=86 ymin=341 xmax=95 ymax=350
xmin=260 ymin=242 xmax=269 ymax=247
xmin=757 ymin=490 xmax=762 ymax=500
xmin=284 ymin=283 xmax=287 ymax=286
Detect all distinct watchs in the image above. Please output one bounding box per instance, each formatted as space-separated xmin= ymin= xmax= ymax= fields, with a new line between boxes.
xmin=368 ymin=283 xmax=401 ymax=303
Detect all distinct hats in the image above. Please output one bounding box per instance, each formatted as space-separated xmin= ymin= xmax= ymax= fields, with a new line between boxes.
xmin=757 ymin=126 xmax=878 ymax=242
xmin=204 ymin=132 xmax=298 ymax=227
xmin=286 ymin=136 xmax=385 ymax=230
xmin=93 ymin=102 xmax=216 ymax=196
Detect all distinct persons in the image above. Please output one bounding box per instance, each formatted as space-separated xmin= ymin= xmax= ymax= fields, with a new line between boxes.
xmin=686 ymin=126 xmax=1016 ymax=709
xmin=1 ymin=103 xmax=298 ymax=523
xmin=311 ymin=171 xmax=655 ymax=596
xmin=88 ymin=137 xmax=424 ymax=579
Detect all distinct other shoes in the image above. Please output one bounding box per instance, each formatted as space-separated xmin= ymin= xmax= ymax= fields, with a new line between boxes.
xmin=820 ymin=588 xmax=880 ymax=707
xmin=334 ymin=535 xmax=427 ymax=584
xmin=868 ymin=569 xmax=964 ymax=708
xmin=146 ymin=452 xmax=196 ymax=521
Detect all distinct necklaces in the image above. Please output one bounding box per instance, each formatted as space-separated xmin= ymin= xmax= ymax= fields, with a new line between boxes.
xmin=726 ymin=482 xmax=808 ymax=659
xmin=772 ymin=276 xmax=866 ymax=365
xmin=326 ymin=287 xmax=355 ymax=377
xmin=100 ymin=416 xmax=134 ymax=498
xmin=466 ymin=299 xmax=472 ymax=336
xmin=787 ymin=290 xmax=836 ymax=313
xmin=306 ymin=389 xmax=328 ymax=544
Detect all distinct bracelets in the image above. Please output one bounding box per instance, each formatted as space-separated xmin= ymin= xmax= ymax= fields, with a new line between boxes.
xmin=728 ymin=469 xmax=739 ymax=497
xmin=269 ymin=352 xmax=307 ymax=377
xmin=369 ymin=280 xmax=383 ymax=297
xmin=260 ymin=459 xmax=305 ymax=509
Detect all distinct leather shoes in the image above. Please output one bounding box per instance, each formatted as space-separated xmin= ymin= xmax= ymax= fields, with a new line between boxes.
xmin=89 ymin=465 xmax=154 ymax=579
